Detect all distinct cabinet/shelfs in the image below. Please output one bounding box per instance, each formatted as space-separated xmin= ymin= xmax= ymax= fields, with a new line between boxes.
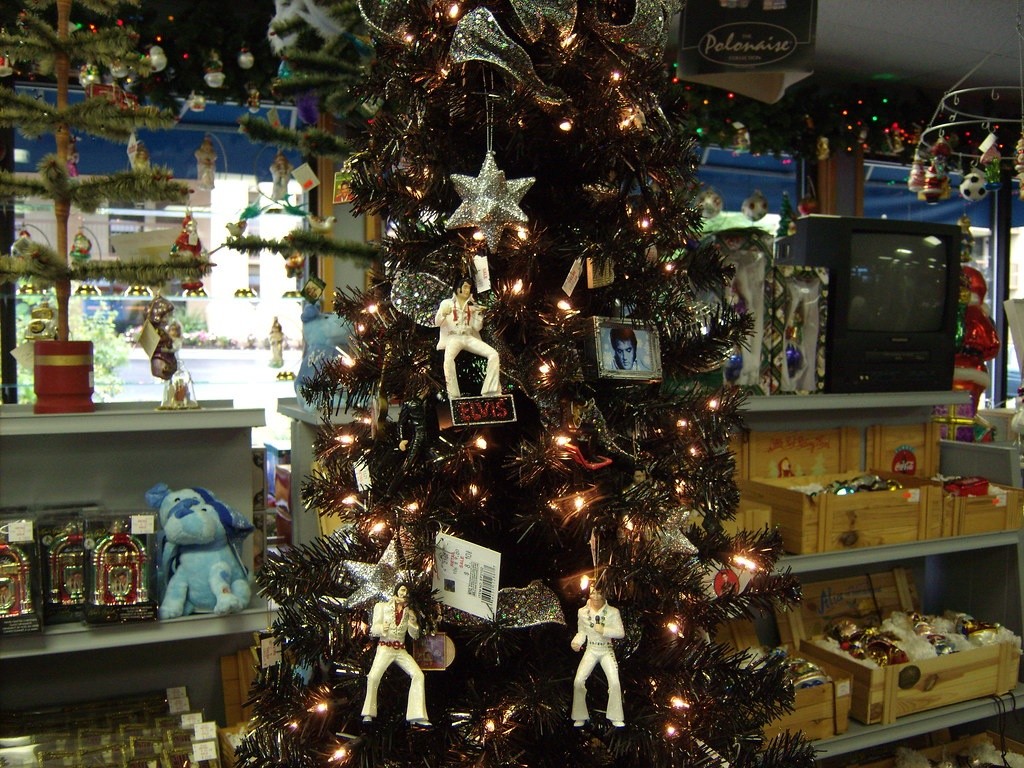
xmin=1 ymin=398 xmax=377 ymax=768
xmin=721 ymin=391 xmax=1024 ymax=768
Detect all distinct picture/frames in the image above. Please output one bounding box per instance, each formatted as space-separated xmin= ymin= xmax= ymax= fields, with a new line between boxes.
xmin=593 ymin=315 xmax=664 ymax=380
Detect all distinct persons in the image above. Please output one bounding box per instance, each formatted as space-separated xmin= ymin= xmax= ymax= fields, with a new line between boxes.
xmin=360 ymin=582 xmax=437 ymax=731
xmin=907 ymin=161 xmax=943 ymax=204
xmin=605 ymin=328 xmax=652 ymax=373
xmin=434 ymin=276 xmax=501 ymax=398
xmin=175 ymin=211 xmax=201 ymax=258
xmin=161 ymin=320 xmax=198 ymax=410
xmin=336 ymin=179 xmax=354 ymax=203
xmin=570 ymin=581 xmax=628 ymax=730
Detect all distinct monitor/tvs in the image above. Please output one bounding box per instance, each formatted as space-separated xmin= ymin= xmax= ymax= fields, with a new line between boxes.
xmin=774 ymin=216 xmax=962 ymax=394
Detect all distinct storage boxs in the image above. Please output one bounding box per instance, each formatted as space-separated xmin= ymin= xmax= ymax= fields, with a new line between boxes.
xmin=773 ymin=567 xmax=1021 ymax=727
xmin=802 ymin=727 xmax=1024 ymax=768
xmin=865 ymin=424 xmax=1024 ymax=540
xmin=704 ymin=613 xmax=855 ymax=740
xmin=682 ymin=493 xmax=773 ymax=562
xmin=722 ymin=425 xmax=943 ymax=555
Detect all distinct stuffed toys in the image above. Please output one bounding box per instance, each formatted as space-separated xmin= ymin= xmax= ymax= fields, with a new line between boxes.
xmin=144 ymin=482 xmax=252 ymax=624
